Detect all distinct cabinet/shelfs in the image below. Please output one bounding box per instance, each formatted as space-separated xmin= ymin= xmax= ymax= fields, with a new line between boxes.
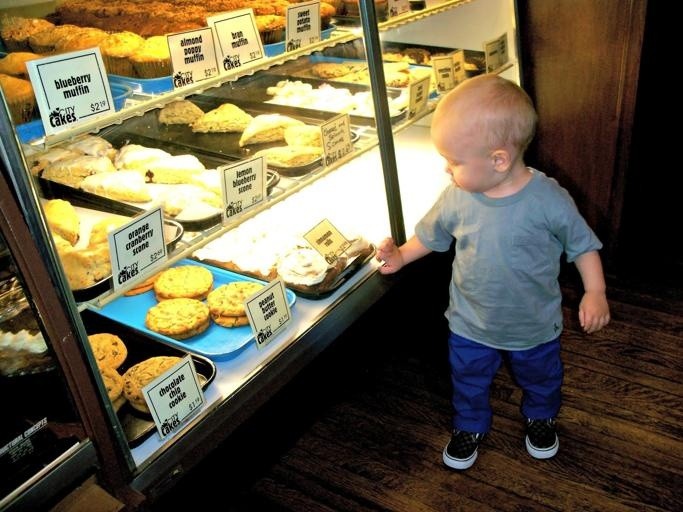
xmin=1 ymin=1 xmax=525 ymax=511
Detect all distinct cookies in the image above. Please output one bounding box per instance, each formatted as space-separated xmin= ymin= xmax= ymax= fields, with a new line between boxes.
xmin=96 ymin=366 xmax=122 ymax=401
xmin=110 ymin=397 xmax=125 ymax=412
xmin=153 ymin=265 xmax=213 ymax=298
xmin=162 ymin=320 xmax=209 ymax=339
xmin=153 ymin=284 xmax=213 ymax=301
xmin=209 ymin=315 xmax=250 ymax=327
xmin=127 ymin=403 xmax=152 ymax=412
xmin=87 ymin=333 xmax=127 ymax=369
xmin=206 ymin=281 xmax=265 ymax=316
xmin=122 ymin=356 xmax=184 ymax=404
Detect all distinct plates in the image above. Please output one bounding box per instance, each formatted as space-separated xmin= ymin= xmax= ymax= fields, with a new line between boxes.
xmin=36 ymin=125 xmax=281 ymax=230
xmin=188 ymin=70 xmax=442 ymax=135
xmin=258 ymin=12 xmax=334 ymax=47
xmin=78 ymin=305 xmax=221 ymax=446
xmin=194 ymin=212 xmax=382 ymax=300
xmin=86 ymin=256 xmax=300 ymax=363
xmin=5 ymin=45 xmax=145 ymax=147
xmin=34 ymin=170 xmax=187 ymax=301
xmin=0 ymin=42 xmax=175 ymax=81
xmin=117 ymin=92 xmax=368 ymax=178
xmin=258 ymin=51 xmax=463 ymax=96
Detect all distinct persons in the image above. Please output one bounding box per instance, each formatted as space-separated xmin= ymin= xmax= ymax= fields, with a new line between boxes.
xmin=377 ymin=74 xmax=611 ymax=469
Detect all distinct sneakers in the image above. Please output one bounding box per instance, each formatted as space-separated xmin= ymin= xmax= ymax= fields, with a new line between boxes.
xmin=527 ymin=418 xmax=559 ymax=458
xmin=443 ymin=429 xmax=484 ymax=470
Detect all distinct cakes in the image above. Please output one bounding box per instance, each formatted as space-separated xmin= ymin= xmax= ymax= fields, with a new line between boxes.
xmin=320 ymin=3 xmax=335 ymax=31
xmin=55 ymin=28 xmax=107 ymax=54
xmin=12 ymin=19 xmax=51 ymax=49
xmin=192 ymin=104 xmax=255 ymax=134
xmin=311 ymin=63 xmax=352 ymax=77
xmin=254 ymin=146 xmax=323 ymax=168
xmin=156 ymin=184 xmax=222 ymax=217
xmin=254 ymin=14 xmax=284 ymax=45
xmin=56 ymin=0 xmax=224 ymax=39
xmin=55 ymin=243 xmax=120 ymax=289
xmin=380 ymin=49 xmax=430 ymax=65
xmin=190 ymin=214 xmax=371 ymax=296
xmin=1 ymin=74 xmax=34 ymax=124
xmin=155 ymin=294 xmax=212 ymax=303
xmin=89 ymin=218 xmax=129 ymax=247
xmin=27 ymin=148 xmax=79 ymax=176
xmin=114 ymin=144 xmax=172 ymax=172
xmin=146 ymin=298 xmax=210 ymax=335
xmin=158 ymin=101 xmax=204 ymax=124
xmin=2 ymin=17 xmax=31 ymax=51
xmin=42 ymin=199 xmax=80 ymax=246
xmin=128 ymin=36 xmax=173 ymax=78
xmin=57 ymin=134 xmax=116 ymax=160
xmin=192 ymin=169 xmax=229 ymax=195
xmin=102 ymin=32 xmax=144 ymax=77
xmin=330 ymin=62 xmax=440 ymax=94
xmin=239 ymin=114 xmax=304 ymax=147
xmin=41 ymin=155 xmax=115 ymax=189
xmin=0 ymin=329 xmax=59 ymax=376
xmin=143 ymin=154 xmax=207 ymax=185
xmin=223 ymin=1 xmax=291 ymax=17
xmin=0 ymin=53 xmax=43 ymax=79
xmin=283 ymin=125 xmax=322 ymax=147
xmin=263 ymin=80 xmax=412 ymax=119
xmin=464 ymin=63 xmax=479 ymax=72
xmin=28 ymin=25 xmax=80 ymax=53
xmin=80 ymin=170 xmax=156 ymax=201
